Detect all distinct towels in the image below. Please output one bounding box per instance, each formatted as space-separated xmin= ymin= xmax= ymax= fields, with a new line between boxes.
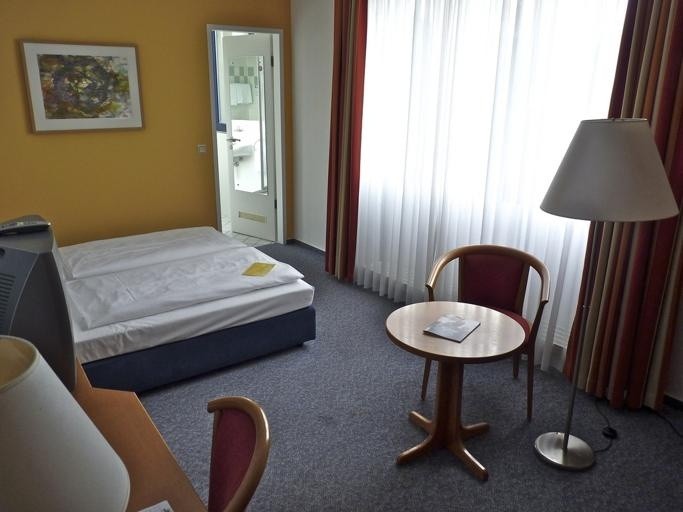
xmin=229 ymin=83 xmax=252 ymax=107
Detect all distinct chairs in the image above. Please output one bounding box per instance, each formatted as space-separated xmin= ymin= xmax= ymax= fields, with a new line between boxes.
xmin=420 ymin=243 xmax=550 ymax=420
xmin=207 ymin=394 xmax=270 ymax=512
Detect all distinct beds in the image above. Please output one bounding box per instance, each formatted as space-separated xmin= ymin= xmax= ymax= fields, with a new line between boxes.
xmin=55 ymin=224 xmax=315 ymax=392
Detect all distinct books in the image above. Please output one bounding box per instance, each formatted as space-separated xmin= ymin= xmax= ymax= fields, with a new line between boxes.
xmin=421 ymin=310 xmax=480 ymax=342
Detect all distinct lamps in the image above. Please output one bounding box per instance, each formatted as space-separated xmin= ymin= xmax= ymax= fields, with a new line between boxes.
xmin=532 ymin=116 xmax=681 ymax=473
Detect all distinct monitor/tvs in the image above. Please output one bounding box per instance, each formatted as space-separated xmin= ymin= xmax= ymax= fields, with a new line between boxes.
xmin=0 ymin=214 xmax=76 ymax=393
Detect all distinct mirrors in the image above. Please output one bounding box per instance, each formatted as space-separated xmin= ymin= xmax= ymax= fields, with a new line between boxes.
xmin=227 ymin=55 xmax=269 ymax=199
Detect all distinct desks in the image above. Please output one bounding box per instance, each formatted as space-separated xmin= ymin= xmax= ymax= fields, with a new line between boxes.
xmin=383 ymin=301 xmax=524 ymax=482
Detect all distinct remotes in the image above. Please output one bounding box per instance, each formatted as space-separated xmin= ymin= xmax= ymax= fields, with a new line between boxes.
xmin=0 ymin=220 xmax=51 ymax=235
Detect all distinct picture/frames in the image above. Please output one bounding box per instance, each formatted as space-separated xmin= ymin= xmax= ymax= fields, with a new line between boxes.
xmin=17 ymin=38 xmax=145 ymax=136
